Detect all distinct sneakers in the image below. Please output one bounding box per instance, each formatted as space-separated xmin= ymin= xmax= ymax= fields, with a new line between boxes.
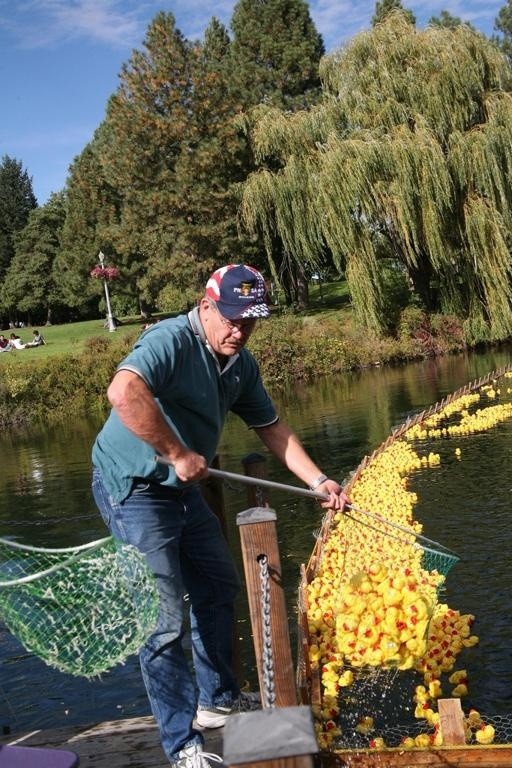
xmin=170 ymin=734 xmax=212 ymax=768
xmin=196 ymin=691 xmax=263 ymax=728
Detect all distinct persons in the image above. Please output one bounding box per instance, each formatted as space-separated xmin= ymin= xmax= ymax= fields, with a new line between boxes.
xmin=142 ymin=318 xmax=161 ymax=329
xmin=0 ymin=319 xmax=47 ymax=353
xmin=89 ymin=262 xmax=354 ymax=768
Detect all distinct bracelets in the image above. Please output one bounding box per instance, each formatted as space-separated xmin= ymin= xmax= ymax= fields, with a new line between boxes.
xmin=310 ymin=473 xmax=328 ymax=491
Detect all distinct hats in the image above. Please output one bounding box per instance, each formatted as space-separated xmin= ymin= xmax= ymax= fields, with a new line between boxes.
xmin=205 ymin=263 xmax=271 ymax=320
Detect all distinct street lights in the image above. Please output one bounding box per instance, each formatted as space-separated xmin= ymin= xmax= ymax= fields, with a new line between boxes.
xmin=99 ymin=251 xmax=116 ymax=332
